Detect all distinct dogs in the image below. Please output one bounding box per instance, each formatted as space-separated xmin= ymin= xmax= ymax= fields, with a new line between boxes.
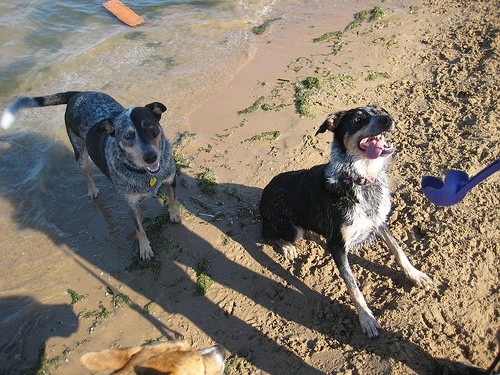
xmin=0 ymin=90 xmax=182 ymax=262
xmin=80 ymin=337 xmax=226 ymax=375
xmin=259 ymin=105 xmax=436 ymax=341
